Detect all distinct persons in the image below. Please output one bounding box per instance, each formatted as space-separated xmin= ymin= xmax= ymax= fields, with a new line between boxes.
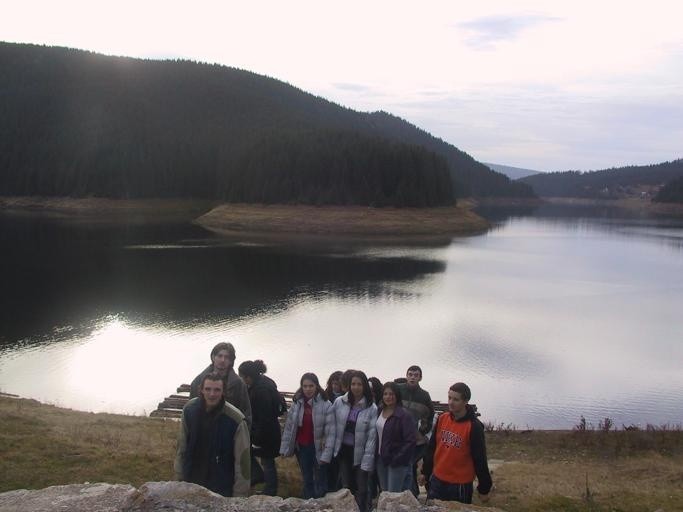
xmin=278 ymin=365 xmax=493 ymax=512
xmin=189 ymin=341 xmax=253 ymax=432
xmin=237 ymin=359 xmax=288 ymax=497
xmin=173 ymin=372 xmax=251 ymax=499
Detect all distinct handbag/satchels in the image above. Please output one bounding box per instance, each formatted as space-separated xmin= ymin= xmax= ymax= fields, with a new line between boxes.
xmin=272 ymin=390 xmax=287 ymax=416
